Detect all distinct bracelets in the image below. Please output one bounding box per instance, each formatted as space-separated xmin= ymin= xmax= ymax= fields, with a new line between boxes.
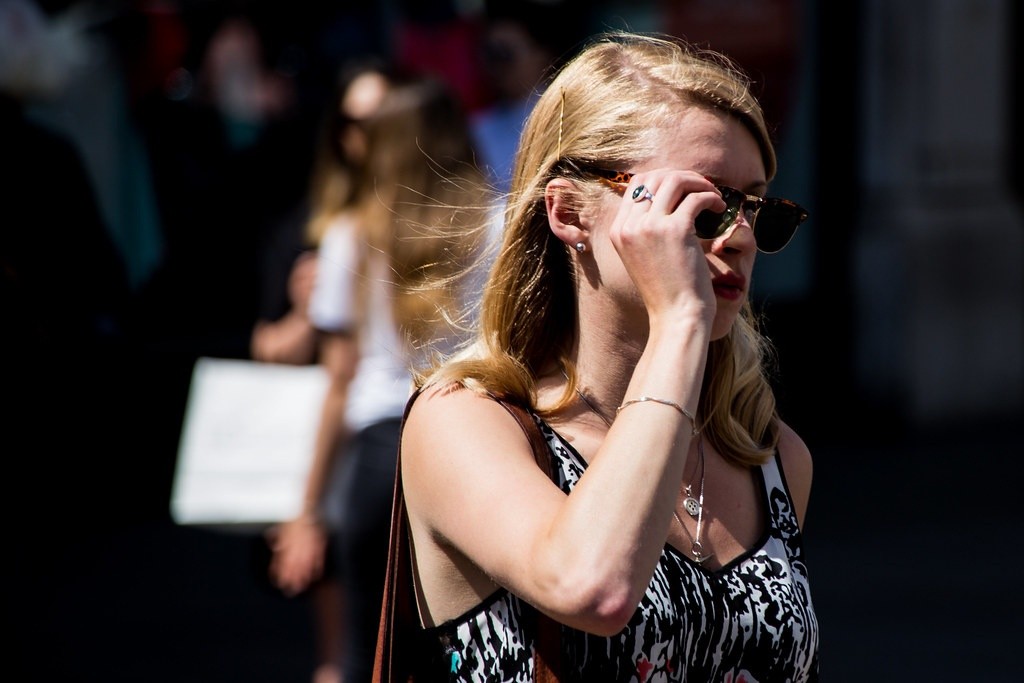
xmin=616 ymin=396 xmax=698 ymax=436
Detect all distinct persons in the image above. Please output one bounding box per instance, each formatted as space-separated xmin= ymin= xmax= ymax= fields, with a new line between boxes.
xmin=399 ymin=31 xmax=817 ymax=683
xmin=1 ymin=0 xmax=552 ymax=683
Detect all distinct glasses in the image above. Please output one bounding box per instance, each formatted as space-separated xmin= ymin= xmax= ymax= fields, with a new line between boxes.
xmin=555 ymin=159 xmax=810 ymax=255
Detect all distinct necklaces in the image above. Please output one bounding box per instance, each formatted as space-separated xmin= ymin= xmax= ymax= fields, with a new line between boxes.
xmin=553 ymin=346 xmax=713 ymax=564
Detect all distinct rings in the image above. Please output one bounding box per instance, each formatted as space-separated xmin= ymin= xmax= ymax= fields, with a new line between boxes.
xmin=633 ymin=185 xmax=655 ymax=203
